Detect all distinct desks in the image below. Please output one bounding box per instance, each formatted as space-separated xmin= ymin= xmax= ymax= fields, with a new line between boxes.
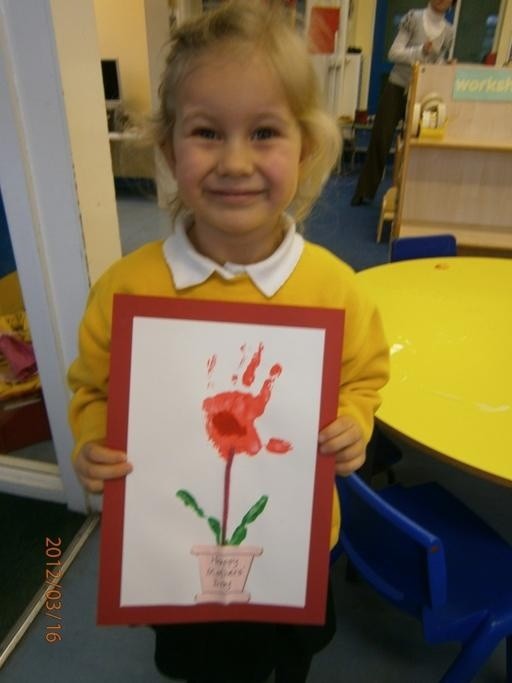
xmin=354 ymin=256 xmax=512 ymax=494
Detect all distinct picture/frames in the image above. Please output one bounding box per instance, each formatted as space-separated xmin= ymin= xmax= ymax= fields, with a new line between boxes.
xmin=94 ymin=293 xmax=349 ymax=629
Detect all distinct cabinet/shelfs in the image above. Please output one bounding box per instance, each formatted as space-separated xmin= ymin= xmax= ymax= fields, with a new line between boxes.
xmin=390 ymin=60 xmax=510 ymax=260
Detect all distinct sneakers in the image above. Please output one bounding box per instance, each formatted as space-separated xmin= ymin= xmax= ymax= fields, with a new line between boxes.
xmin=351 ymin=196 xmax=367 ymax=206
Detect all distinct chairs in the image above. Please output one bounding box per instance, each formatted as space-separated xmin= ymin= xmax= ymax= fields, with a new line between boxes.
xmin=330 ymin=461 xmax=509 ymax=683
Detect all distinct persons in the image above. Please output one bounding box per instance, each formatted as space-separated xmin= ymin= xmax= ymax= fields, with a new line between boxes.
xmin=65 ymin=1 xmax=393 ymax=683
xmin=353 ymin=0 xmax=455 ymax=206
